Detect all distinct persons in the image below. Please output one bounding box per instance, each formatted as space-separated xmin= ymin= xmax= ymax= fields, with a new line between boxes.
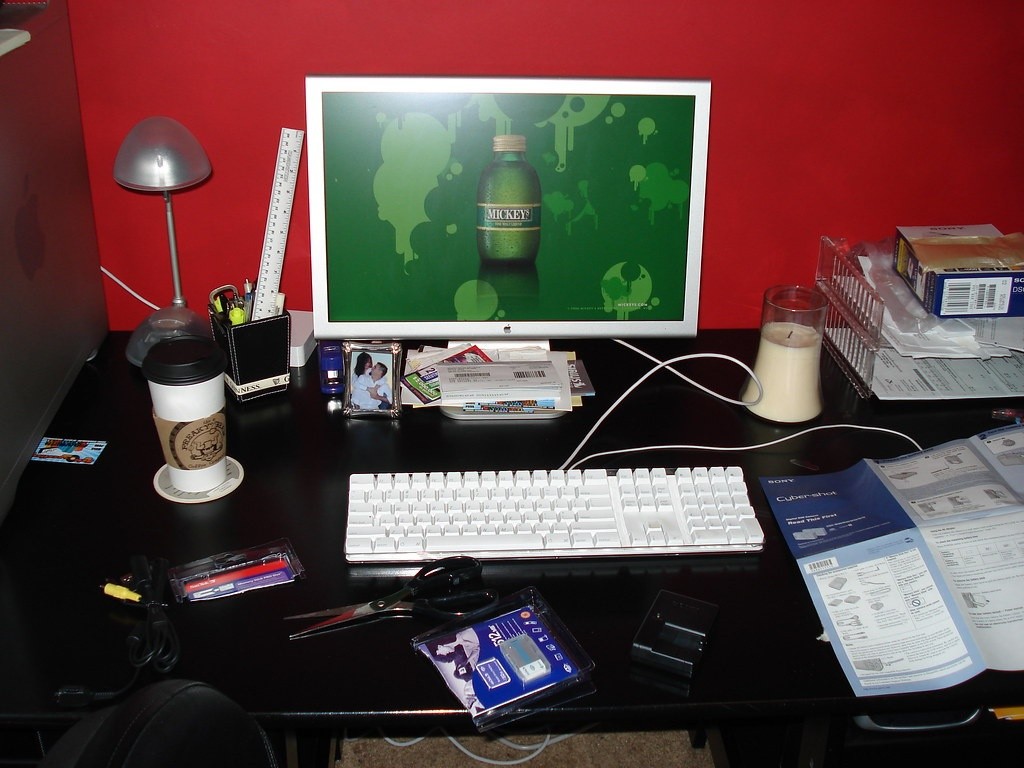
xmin=351 ymin=352 xmax=392 ymax=409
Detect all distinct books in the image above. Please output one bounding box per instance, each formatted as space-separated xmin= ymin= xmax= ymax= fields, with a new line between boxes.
xmin=400 ymin=345 xmax=595 ymax=414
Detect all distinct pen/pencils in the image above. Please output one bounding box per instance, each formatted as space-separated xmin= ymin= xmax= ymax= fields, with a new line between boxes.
xmin=215 ymin=275 xmax=254 ymax=326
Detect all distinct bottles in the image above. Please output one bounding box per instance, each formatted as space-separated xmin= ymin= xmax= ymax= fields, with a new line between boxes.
xmin=476 ymin=135 xmax=541 ymax=265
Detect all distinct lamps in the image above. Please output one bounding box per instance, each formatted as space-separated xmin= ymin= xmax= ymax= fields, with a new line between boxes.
xmin=113 ymin=116 xmax=214 ymax=366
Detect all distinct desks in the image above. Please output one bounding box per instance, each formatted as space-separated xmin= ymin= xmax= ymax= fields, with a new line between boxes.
xmin=0 ymin=329 xmax=1024 ymax=768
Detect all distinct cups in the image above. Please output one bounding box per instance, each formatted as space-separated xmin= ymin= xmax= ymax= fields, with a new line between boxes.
xmin=738 ymin=285 xmax=830 ymax=424
xmin=143 ymin=334 xmax=229 ymax=491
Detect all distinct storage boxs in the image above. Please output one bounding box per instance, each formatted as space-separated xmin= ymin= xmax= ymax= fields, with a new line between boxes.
xmin=895 ymin=224 xmax=1024 ymax=319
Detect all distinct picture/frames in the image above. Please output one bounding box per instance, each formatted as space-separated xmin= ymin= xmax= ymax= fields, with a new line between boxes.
xmin=340 ymin=341 xmax=403 ymax=421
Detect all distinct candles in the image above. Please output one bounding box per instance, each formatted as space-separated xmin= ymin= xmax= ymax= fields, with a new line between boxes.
xmin=741 ymin=282 xmax=831 ymax=425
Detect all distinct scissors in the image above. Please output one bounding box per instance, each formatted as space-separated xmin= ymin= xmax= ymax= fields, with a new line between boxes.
xmin=286 ymin=555 xmax=501 ymax=641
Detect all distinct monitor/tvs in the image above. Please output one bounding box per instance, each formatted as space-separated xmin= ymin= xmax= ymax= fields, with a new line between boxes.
xmin=305 ymin=74 xmax=711 ymax=356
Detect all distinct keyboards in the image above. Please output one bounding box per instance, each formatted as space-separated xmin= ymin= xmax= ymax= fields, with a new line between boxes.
xmin=345 ymin=466 xmax=765 ymax=564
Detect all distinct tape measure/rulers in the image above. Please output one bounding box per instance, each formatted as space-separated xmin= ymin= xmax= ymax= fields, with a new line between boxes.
xmin=252 ymin=126 xmax=306 ymax=319
